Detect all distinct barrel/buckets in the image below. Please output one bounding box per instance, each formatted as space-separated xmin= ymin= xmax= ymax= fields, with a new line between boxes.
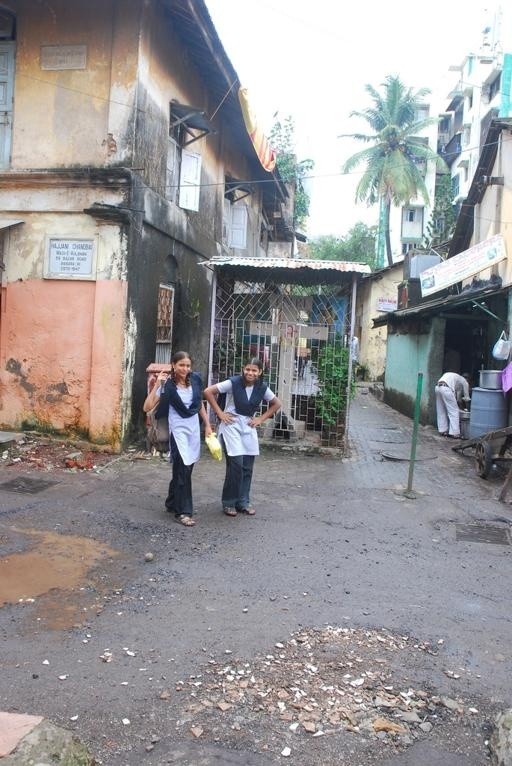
xmin=469 ymin=386 xmax=507 ymax=440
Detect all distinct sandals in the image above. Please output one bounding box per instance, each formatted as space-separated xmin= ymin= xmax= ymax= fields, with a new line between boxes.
xmin=174 ymin=514 xmax=196 ymax=526
xmin=224 ymin=502 xmax=256 ymax=516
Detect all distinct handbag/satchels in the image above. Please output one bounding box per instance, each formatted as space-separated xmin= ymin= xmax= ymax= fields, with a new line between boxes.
xmin=492 ymin=330 xmax=512 ymax=360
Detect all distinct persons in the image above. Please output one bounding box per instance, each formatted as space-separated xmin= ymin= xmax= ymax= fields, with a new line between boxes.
xmin=287 ymin=326 xmax=293 ymax=338
xmin=203 ymin=358 xmax=281 ymax=516
xmin=346 ymin=326 xmax=360 ymax=364
xmin=434 ymin=371 xmax=471 ymax=440
xmin=143 ymin=351 xmax=212 ymax=526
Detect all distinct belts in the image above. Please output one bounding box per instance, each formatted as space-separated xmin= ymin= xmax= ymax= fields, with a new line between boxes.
xmin=435 ymin=384 xmax=449 ymax=386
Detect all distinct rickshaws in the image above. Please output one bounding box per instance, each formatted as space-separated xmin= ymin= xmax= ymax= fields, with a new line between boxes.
xmin=449 ymin=425 xmax=512 ymax=478
